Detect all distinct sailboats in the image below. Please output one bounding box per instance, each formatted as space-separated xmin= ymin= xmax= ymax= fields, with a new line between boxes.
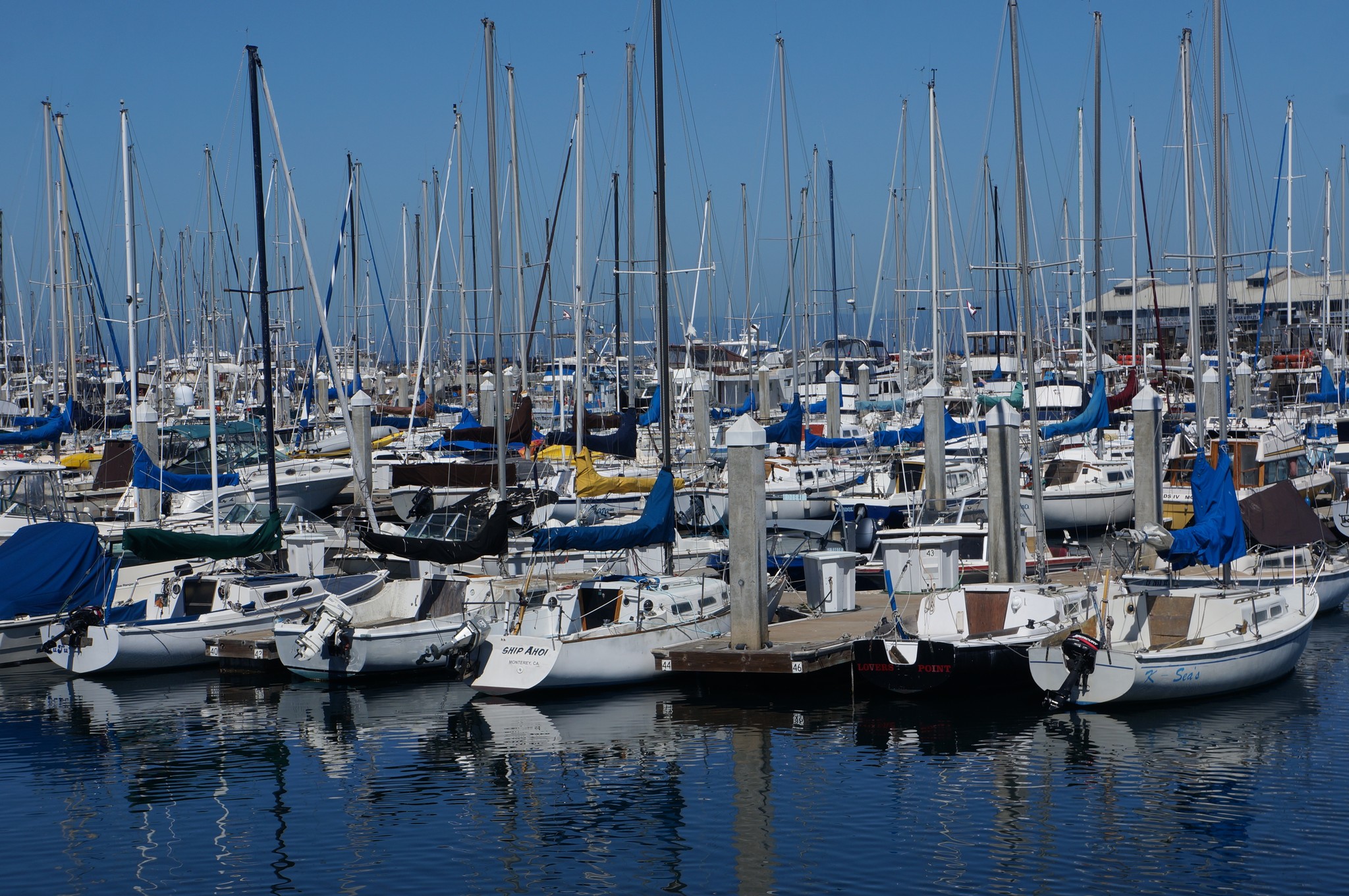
xmin=0 ymin=1 xmax=1349 ymax=703
